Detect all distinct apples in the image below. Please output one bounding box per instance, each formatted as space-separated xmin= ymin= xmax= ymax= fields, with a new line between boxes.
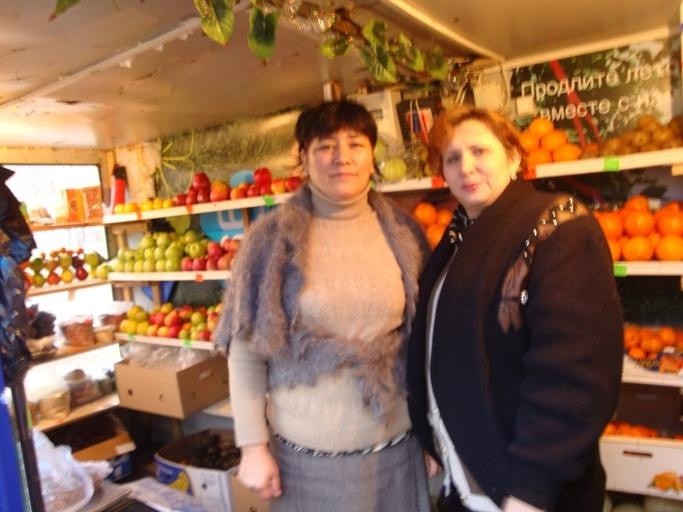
xmin=119 ymin=302 xmax=223 ymax=341
xmin=114 ymin=167 xmax=302 ymax=214
xmin=30 ymin=229 xmax=241 ymax=288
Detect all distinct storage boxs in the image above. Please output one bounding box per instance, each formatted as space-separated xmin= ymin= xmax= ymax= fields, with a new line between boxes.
xmin=54 ymin=409 xmax=265 ymax=509
xmin=113 ymin=346 xmax=230 ymax=420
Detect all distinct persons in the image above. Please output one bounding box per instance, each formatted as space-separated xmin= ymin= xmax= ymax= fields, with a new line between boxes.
xmin=213 ymin=100 xmax=444 ymax=512
xmin=408 ymin=104 xmax=624 ymax=511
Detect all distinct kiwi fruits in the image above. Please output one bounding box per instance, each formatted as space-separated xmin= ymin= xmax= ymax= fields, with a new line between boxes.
xmin=578 ymin=114 xmax=683 ymax=160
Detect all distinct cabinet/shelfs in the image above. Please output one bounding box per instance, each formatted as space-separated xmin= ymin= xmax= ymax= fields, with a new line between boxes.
xmin=372 ymin=144 xmax=683 ymax=512
xmin=13 ymin=218 xmax=122 ymax=432
xmin=104 ymin=192 xmax=293 ymax=415
